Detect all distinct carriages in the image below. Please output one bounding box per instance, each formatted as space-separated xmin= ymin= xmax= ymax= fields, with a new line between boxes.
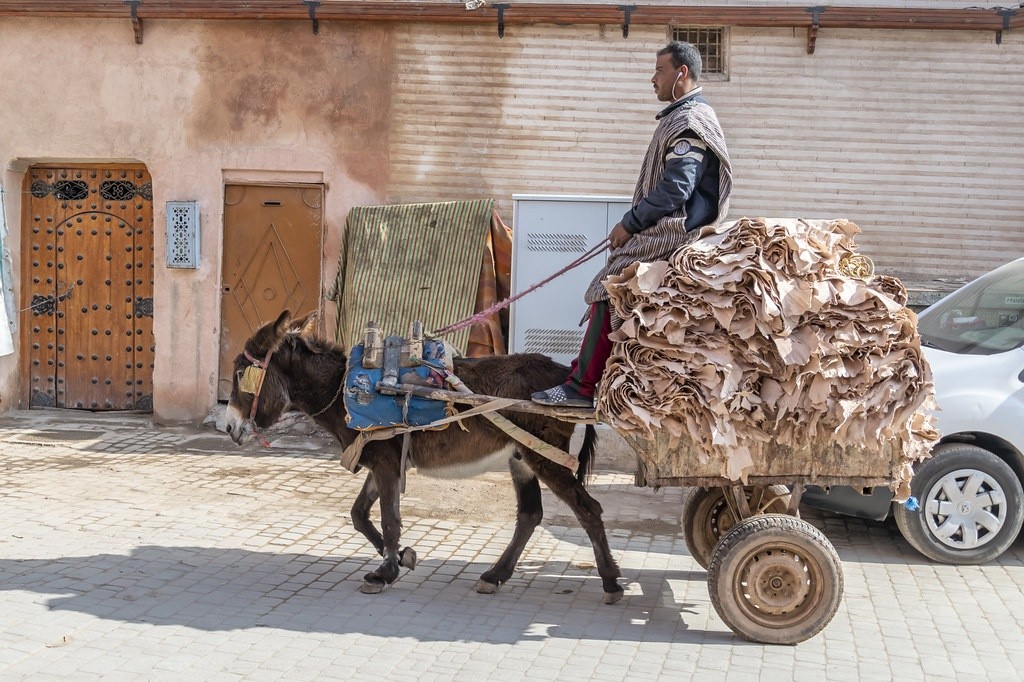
xmin=214 ymin=220 xmax=938 ymax=645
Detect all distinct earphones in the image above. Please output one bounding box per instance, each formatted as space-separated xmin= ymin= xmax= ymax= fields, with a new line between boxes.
xmin=676 ymin=72 xmax=683 ymax=79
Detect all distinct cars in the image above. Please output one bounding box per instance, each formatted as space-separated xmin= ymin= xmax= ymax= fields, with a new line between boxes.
xmin=787 ymin=255 xmax=1024 ymax=567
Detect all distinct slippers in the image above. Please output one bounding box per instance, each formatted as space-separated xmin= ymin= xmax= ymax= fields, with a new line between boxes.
xmin=531 ymin=382 xmax=594 ymax=408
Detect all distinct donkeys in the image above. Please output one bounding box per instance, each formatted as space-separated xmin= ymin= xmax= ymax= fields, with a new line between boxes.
xmin=224 ymin=309 xmax=626 ymax=604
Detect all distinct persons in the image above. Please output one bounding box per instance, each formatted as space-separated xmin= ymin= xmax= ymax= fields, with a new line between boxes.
xmin=530 ymin=41 xmax=733 ymax=407
